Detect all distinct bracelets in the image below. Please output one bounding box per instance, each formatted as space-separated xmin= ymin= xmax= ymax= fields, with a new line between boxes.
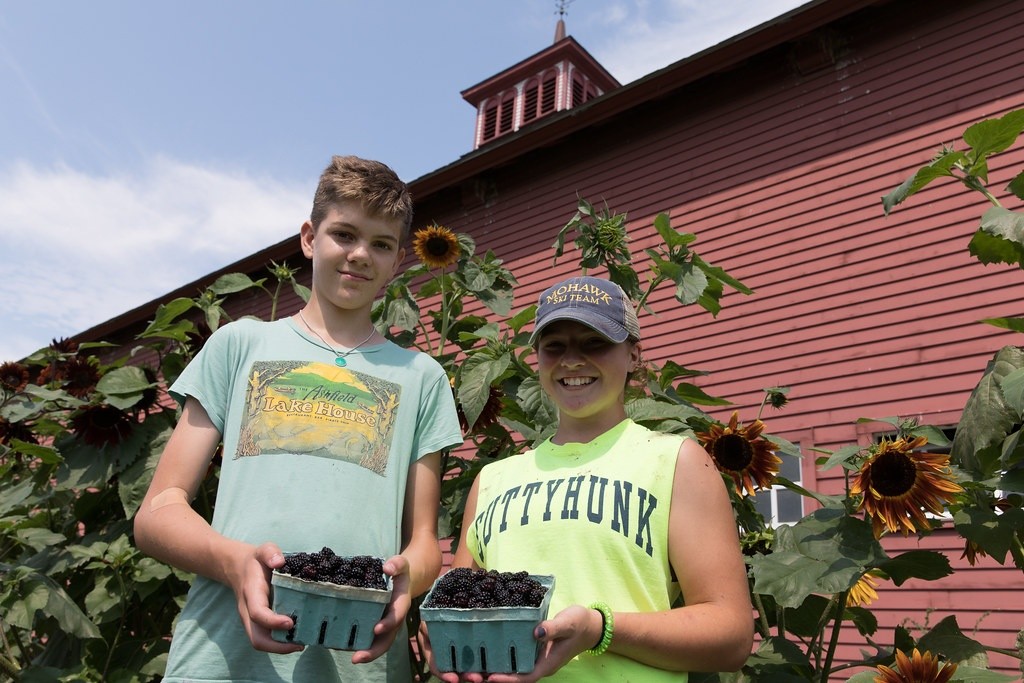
xmin=587 ymin=601 xmax=614 ymax=656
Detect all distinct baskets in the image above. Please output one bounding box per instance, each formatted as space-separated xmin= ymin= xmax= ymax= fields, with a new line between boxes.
xmin=271 ymin=552 xmax=395 ymax=651
xmin=419 ymin=567 xmax=555 ymax=674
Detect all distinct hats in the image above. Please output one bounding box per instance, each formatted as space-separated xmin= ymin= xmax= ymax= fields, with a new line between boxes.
xmin=528 ymin=276 xmax=641 ymax=351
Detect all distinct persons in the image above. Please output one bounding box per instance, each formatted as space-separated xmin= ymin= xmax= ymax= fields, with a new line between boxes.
xmin=133 ymin=154 xmax=463 ymax=683
xmin=416 ymin=276 xmax=755 ymax=683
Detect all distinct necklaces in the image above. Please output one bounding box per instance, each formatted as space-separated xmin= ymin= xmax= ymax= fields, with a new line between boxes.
xmin=300 ymin=310 xmax=375 ymax=367
